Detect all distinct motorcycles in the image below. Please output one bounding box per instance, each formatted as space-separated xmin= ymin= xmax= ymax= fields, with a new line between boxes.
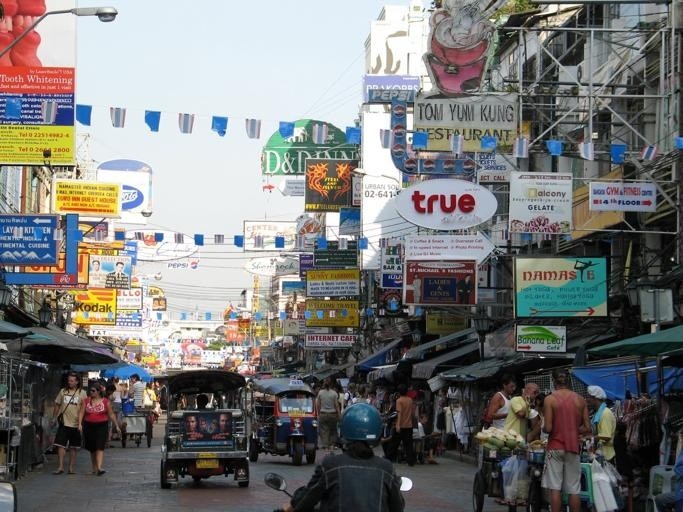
xmin=156 ymin=370 xmax=254 ymax=490
xmin=150 ymin=398 xmax=162 ymax=419
xmin=244 ymin=371 xmax=319 ymax=467
xmin=262 ymin=470 xmax=416 ymax=512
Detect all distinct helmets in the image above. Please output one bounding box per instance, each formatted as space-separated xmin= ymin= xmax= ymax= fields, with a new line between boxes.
xmin=338 ymin=402 xmax=384 ymax=443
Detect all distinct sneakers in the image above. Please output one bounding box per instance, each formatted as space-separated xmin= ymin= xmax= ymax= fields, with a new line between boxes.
xmin=428 ymin=458 xmax=440 ymax=466
xmin=92 ymin=470 xmax=106 ymax=476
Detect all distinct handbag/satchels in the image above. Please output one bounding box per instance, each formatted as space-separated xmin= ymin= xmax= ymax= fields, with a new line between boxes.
xmin=57 ymin=413 xmax=65 ymax=429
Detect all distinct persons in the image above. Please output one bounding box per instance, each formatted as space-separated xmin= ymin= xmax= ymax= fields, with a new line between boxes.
xmin=456 ymin=274 xmax=473 ymax=305
xmin=486 ymin=368 xmax=616 ymax=512
xmin=308 ymin=377 xmax=439 ymax=465
xmin=281 ymin=404 xmax=405 ymax=512
xmin=655 ymin=448 xmax=683 ymax=512
xmin=97 ymin=373 xmax=167 ymax=447
xmin=196 ymin=394 xmax=209 ymax=409
xmin=105 ymin=261 xmax=129 ymax=288
xmin=88 ymin=259 xmax=106 ymax=288
xmin=411 ymin=272 xmax=421 ymax=303
xmin=212 ymin=413 xmax=233 ymax=438
xmin=185 ymin=415 xmax=205 ymax=442
xmin=48 ymin=372 xmax=121 ymax=476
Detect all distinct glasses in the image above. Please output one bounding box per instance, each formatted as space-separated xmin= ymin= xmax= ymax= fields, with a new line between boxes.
xmin=88 ymin=389 xmax=98 ymax=393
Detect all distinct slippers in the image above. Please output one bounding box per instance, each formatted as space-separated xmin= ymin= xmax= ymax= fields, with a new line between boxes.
xmin=67 ymin=470 xmax=77 ymax=475
xmin=52 ymin=468 xmax=64 ymax=475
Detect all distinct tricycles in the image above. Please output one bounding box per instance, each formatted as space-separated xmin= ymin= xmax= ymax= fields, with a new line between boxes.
xmin=120 ymin=412 xmax=154 ymax=449
xmin=469 ymin=439 xmax=546 ymax=511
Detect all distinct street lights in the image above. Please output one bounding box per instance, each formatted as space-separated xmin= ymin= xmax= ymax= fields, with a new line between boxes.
xmin=0 ymin=4 xmax=123 ymax=66
xmin=223 ymin=305 xmax=257 ymax=344
xmin=82 ymin=207 xmax=155 ymax=238
xmin=254 ymin=292 xmax=286 ymax=348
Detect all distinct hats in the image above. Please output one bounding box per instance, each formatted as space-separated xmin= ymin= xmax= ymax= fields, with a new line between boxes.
xmin=551 ymin=368 xmax=569 ymax=379
xmin=586 ymin=384 xmax=608 ymax=401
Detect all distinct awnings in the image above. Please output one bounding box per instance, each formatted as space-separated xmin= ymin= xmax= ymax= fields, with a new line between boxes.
xmin=368 ymin=361 xmax=419 ymax=384
xmin=440 ymin=361 xmax=525 ymax=382
